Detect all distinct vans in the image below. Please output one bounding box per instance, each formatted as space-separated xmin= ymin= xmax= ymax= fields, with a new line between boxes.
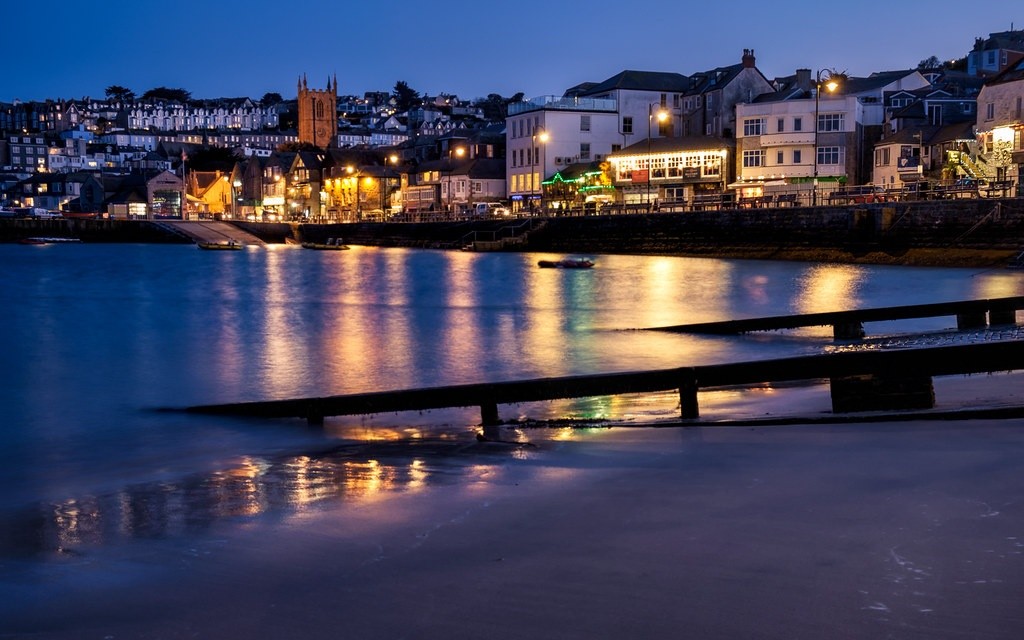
xmin=472 ymin=202 xmax=512 ymax=219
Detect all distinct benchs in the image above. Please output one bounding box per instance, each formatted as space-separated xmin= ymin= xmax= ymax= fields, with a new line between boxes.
xmin=521 ymin=179 xmax=1016 ymax=216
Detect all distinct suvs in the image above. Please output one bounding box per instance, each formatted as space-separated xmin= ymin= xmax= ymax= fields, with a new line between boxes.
xmin=28 ymin=208 xmax=56 ymax=220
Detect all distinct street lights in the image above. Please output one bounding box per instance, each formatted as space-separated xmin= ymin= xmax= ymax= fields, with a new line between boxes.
xmin=448 ymin=146 xmax=466 ymax=219
xmin=347 ymin=165 xmax=360 ymax=222
xmin=384 ymin=155 xmax=398 ymax=222
xmin=813 ymin=68 xmax=839 ymax=206
xmin=647 ymin=102 xmax=668 ymax=213
xmin=529 ymin=125 xmax=549 ymax=216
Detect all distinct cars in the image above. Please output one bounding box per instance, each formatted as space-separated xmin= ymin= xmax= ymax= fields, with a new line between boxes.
xmin=298 ymin=216 xmax=308 ymax=223
xmin=848 ymin=187 xmax=893 ymax=205
xmin=262 ymin=209 xmax=283 ymax=223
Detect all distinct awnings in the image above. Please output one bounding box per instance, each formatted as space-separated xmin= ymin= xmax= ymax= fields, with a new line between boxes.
xmin=727 ymin=180 xmax=764 ymax=189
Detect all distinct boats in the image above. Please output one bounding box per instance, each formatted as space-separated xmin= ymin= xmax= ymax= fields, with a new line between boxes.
xmin=302 ymin=242 xmax=350 ymax=250
xmin=20 ymin=237 xmax=45 ymax=245
xmin=537 ymin=257 xmax=595 ymax=269
xmin=198 ymin=240 xmax=244 ymax=250
